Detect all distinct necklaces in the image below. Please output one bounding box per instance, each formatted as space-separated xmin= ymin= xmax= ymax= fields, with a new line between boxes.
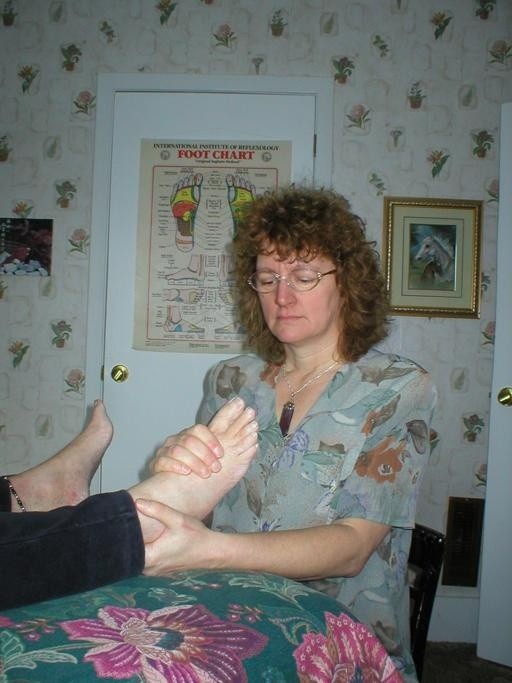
xmin=271 ymin=360 xmax=344 ymax=437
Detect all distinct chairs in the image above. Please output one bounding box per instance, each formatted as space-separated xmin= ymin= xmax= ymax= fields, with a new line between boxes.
xmin=402 ymin=523 xmax=446 ymax=682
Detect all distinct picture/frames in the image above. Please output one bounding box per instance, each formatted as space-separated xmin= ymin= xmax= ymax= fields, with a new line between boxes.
xmin=381 ymin=194 xmax=483 ymax=320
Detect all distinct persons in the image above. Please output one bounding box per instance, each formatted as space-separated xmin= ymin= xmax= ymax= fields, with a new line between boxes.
xmin=0 ymin=395 xmax=259 ymax=619
xmin=138 ymin=182 xmax=437 ymax=683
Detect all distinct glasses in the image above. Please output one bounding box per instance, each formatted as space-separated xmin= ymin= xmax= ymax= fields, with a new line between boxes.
xmin=247 ymin=268 xmax=339 ymax=294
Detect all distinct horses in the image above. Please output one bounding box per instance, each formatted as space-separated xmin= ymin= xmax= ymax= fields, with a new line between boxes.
xmin=414 ymin=232 xmax=454 ymax=281
